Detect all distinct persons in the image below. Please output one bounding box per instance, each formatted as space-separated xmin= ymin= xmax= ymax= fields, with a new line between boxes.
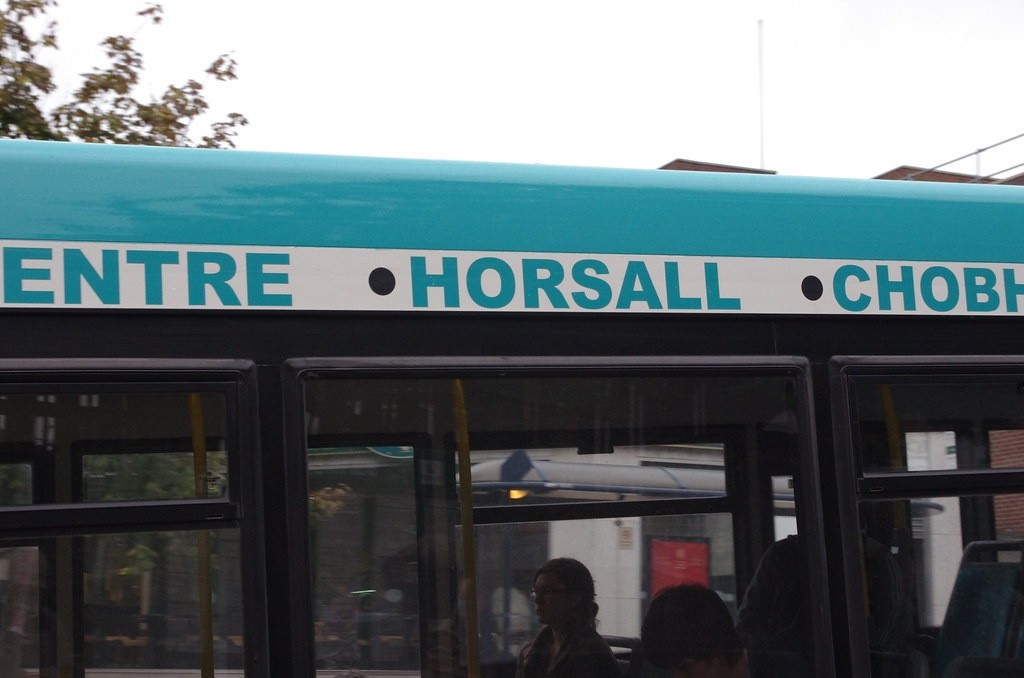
xmin=319 ymin=541 xmax=426 ymax=672
xmin=639 ymin=582 xmax=752 ymax=678
xmin=734 ymin=449 xmax=906 ymax=678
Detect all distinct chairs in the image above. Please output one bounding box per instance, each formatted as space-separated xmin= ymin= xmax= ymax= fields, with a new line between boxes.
xmin=924 ymin=539 xmax=1024 ymax=678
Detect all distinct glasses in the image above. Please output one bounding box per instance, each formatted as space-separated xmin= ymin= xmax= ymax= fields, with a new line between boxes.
xmin=529 ymin=586 xmax=566 ymax=601
